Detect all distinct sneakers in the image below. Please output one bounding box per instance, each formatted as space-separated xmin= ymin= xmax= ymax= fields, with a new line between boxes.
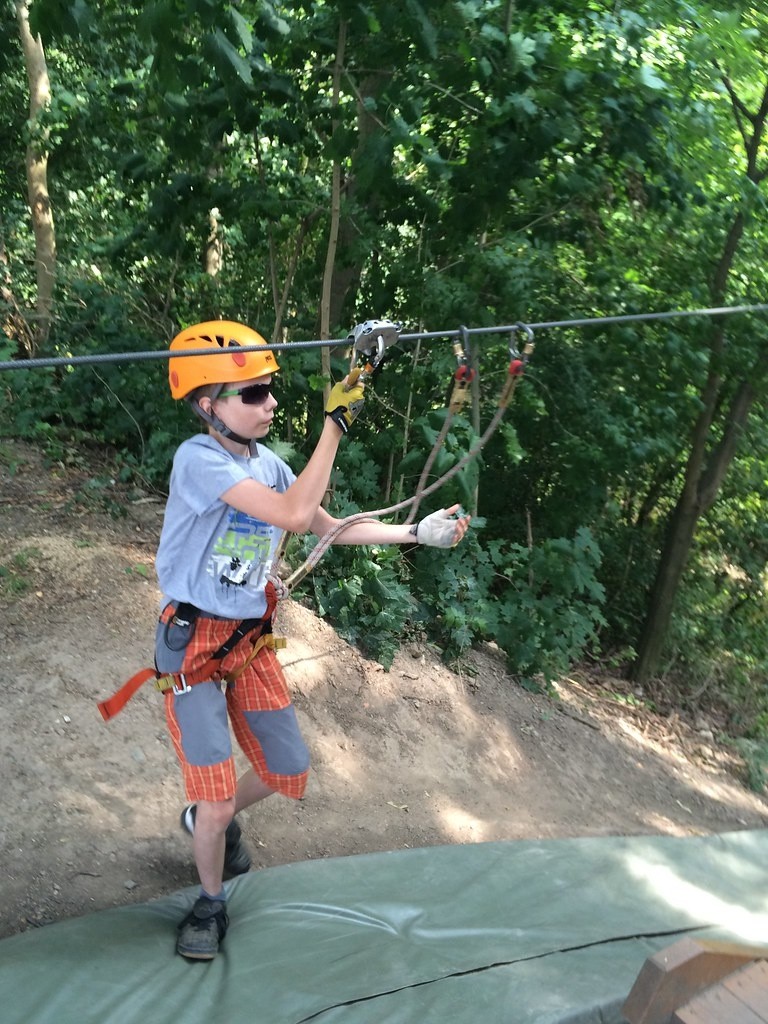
xmin=180 ymin=801 xmax=253 ymax=876
xmin=176 ymin=894 xmax=229 ymax=962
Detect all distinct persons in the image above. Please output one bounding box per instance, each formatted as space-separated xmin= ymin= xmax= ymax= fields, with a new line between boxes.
xmin=153 ymin=320 xmax=471 ymax=961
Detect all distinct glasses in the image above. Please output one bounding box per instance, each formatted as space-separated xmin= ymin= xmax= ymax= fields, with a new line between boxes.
xmin=218 ymin=377 xmax=274 ymax=404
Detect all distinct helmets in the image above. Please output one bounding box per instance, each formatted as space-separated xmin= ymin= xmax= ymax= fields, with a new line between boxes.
xmin=167 ymin=320 xmax=280 ymax=461
